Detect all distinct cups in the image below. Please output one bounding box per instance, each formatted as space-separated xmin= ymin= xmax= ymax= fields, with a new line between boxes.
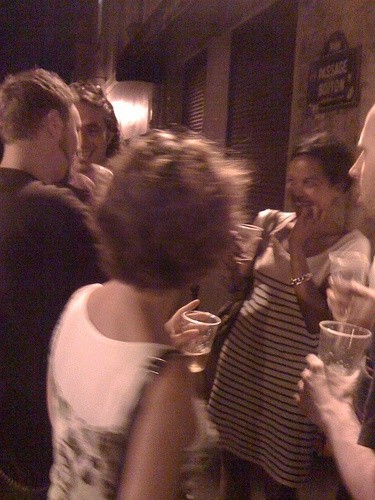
xmin=318 ymin=321 xmax=372 ymax=376
xmin=327 ymin=250 xmax=367 ymax=282
xmin=181 ymin=310 xmax=221 ymax=372
xmin=235 ymin=223 xmax=263 ymax=268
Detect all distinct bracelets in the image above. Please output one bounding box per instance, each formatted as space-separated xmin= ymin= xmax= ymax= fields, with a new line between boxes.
xmin=289 ymin=272 xmax=314 ymax=286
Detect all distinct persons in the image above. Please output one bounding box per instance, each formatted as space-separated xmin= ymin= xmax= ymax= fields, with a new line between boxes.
xmin=0 ymin=68 xmax=374 ymax=499
